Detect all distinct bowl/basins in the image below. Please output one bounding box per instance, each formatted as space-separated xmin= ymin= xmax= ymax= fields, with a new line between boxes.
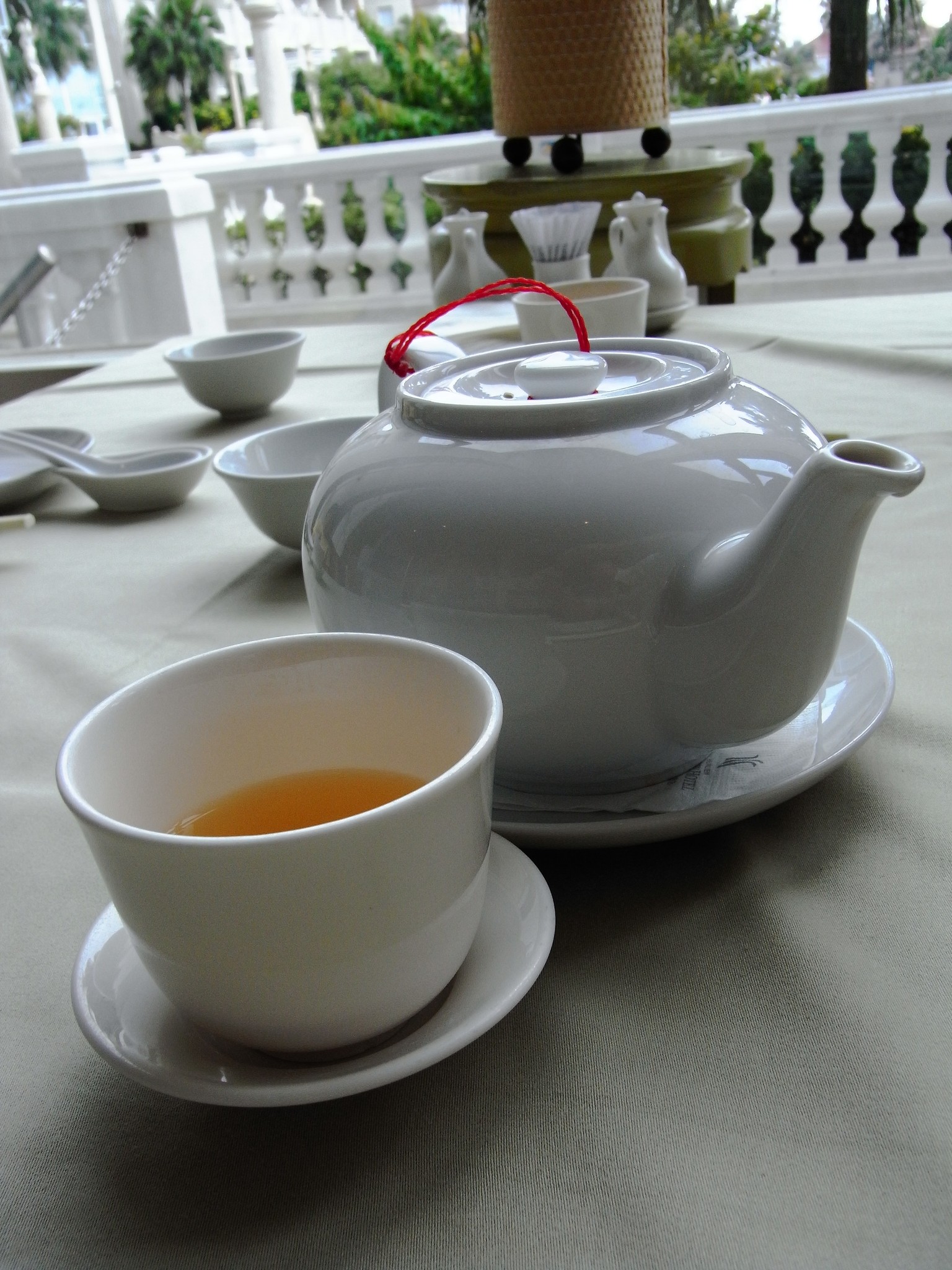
xmin=213 ymin=415 xmax=372 ymax=550
xmin=52 ymin=445 xmax=212 ymax=510
xmin=164 ymin=330 xmax=303 ymax=421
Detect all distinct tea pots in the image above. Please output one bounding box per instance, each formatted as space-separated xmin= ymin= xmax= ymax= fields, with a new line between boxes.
xmin=602 ymin=191 xmax=687 ymax=312
xmin=300 ymin=330 xmax=924 ymax=794
xmin=434 ymin=208 xmax=512 ymax=307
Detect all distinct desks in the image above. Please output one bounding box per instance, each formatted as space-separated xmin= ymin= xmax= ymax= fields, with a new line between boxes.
xmin=0 ymin=289 xmax=952 ymax=1270
xmin=421 ymin=147 xmax=755 ymax=308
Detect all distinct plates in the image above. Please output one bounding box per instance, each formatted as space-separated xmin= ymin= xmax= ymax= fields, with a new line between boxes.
xmin=647 ymin=297 xmax=696 ymax=331
xmin=71 ymin=829 xmax=557 ymax=1107
xmin=0 ymin=428 xmax=94 ymax=506
xmin=484 ymin=614 xmax=895 ymax=849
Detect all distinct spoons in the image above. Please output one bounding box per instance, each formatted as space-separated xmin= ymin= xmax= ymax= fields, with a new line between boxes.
xmin=1 ymin=430 xmax=202 ymax=475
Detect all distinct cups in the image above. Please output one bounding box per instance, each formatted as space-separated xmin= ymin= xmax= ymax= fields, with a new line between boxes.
xmin=54 ymin=633 xmax=503 ymax=1061
xmin=511 ymin=277 xmax=649 ymax=344
xmin=531 ymin=253 xmax=590 ymax=285
